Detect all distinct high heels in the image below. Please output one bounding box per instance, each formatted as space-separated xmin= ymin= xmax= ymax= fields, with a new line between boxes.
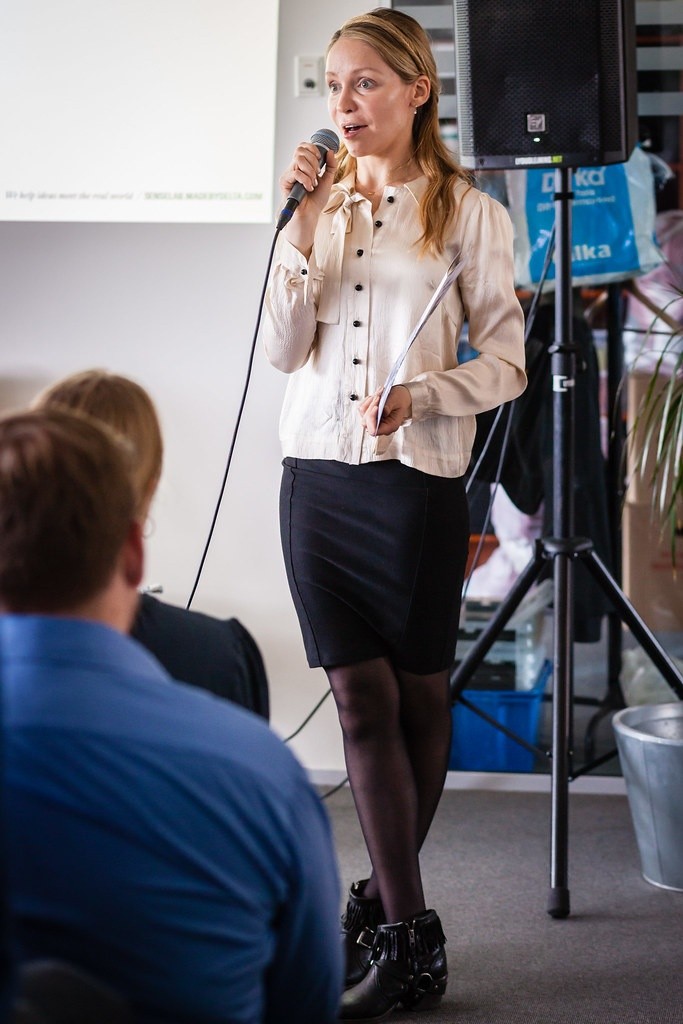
xmin=338 ymin=909 xmax=449 ymax=1024
xmin=340 ymin=878 xmax=388 ymax=987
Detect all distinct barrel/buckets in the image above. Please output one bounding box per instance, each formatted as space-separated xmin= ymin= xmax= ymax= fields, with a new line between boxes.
xmin=612 ymin=702 xmax=683 ymax=892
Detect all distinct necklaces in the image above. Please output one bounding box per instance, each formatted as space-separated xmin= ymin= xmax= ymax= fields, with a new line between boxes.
xmin=355 ymin=178 xmax=403 ymax=195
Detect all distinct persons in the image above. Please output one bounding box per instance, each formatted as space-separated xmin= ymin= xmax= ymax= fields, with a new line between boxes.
xmin=0 ymin=370 xmax=269 ymax=721
xmin=0 ymin=412 xmax=343 ymax=1024
xmin=261 ymin=7 xmax=527 ymax=1023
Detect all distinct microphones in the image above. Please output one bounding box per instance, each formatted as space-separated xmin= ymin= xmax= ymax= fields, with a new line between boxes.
xmin=277 ymin=129 xmax=339 ymax=230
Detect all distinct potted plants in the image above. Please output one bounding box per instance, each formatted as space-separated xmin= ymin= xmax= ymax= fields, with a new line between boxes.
xmin=612 ymin=245 xmax=683 ymax=895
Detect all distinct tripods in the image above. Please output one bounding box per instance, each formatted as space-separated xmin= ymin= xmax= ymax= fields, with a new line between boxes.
xmin=446 ymin=170 xmax=681 ymax=920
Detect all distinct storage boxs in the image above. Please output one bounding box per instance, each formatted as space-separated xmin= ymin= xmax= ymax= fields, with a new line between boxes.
xmin=445 ymin=575 xmax=555 ymax=771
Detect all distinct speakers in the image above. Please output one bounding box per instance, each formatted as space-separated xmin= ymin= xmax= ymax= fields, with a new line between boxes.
xmin=453 ymin=0 xmax=639 ymax=171
